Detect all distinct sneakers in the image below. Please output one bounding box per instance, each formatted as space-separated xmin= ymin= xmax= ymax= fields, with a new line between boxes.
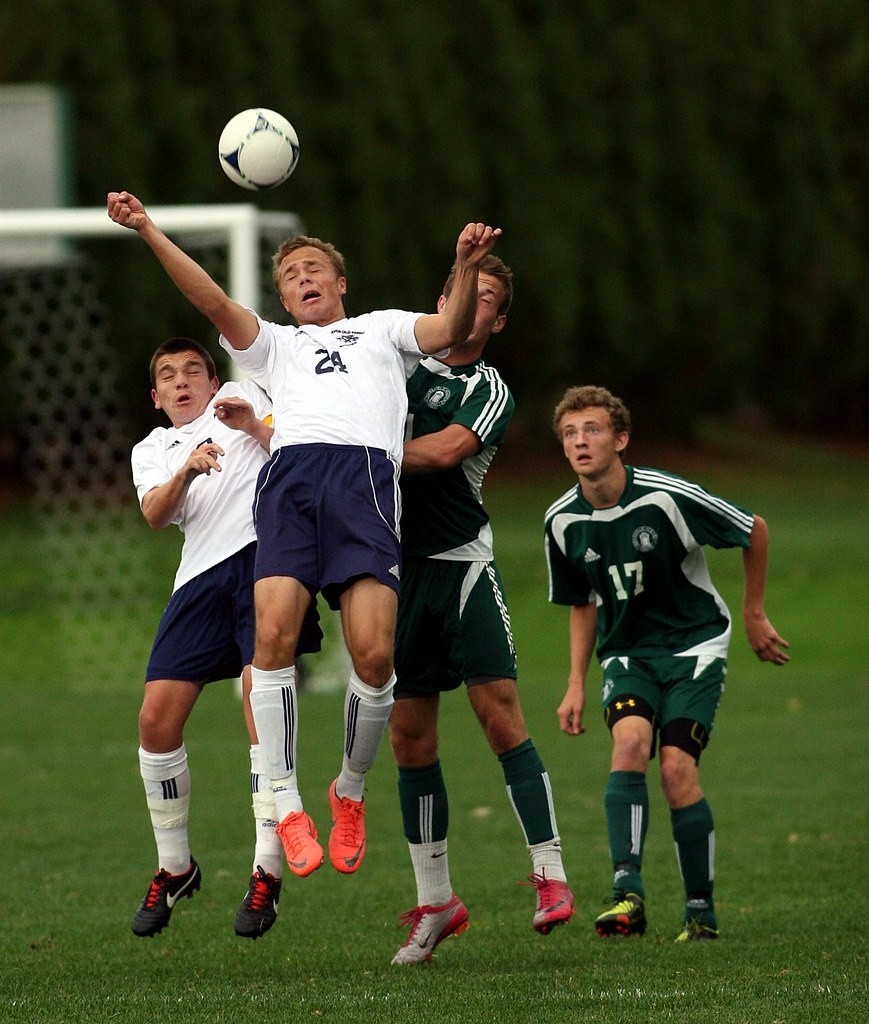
xmin=515 ymin=870 xmax=577 ymax=934
xmin=672 ymin=924 xmax=722 ymax=946
xmin=132 ymin=856 xmax=203 ymax=938
xmin=232 ymin=864 xmax=285 ymax=937
xmin=594 ymin=894 xmax=649 ymax=936
xmin=391 ymin=894 xmax=468 ymax=970
xmin=277 ymin=806 xmax=324 ymax=880
xmin=327 ymin=779 xmax=369 ymax=872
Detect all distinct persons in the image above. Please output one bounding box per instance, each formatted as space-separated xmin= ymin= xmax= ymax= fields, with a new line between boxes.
xmin=541 ymin=383 xmax=793 ymax=943
xmin=370 ymin=248 xmax=582 ymax=973
xmin=106 ymin=186 xmax=506 ymax=881
xmin=124 ymin=337 xmax=323 ymax=938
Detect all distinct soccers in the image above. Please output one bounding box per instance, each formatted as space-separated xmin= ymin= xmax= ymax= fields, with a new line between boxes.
xmin=216 ymin=105 xmax=301 ymax=194
